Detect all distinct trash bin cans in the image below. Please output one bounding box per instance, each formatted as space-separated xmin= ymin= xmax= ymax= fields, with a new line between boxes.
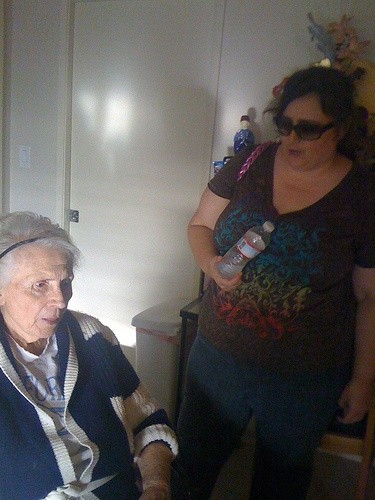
xmin=131 ymin=297 xmax=195 ymax=426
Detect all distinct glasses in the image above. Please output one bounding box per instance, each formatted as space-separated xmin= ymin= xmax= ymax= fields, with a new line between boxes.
xmin=272 ymin=112 xmax=347 ymax=140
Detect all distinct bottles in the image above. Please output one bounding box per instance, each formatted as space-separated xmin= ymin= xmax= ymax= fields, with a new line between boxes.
xmin=216 ymin=221 xmax=275 ymax=280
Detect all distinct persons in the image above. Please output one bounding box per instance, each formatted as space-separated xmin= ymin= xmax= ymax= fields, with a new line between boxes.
xmin=234 ymin=115 xmax=255 ymax=156
xmin=0 ymin=210 xmax=179 ymax=500
xmin=170 ymin=66 xmax=375 ymax=500
